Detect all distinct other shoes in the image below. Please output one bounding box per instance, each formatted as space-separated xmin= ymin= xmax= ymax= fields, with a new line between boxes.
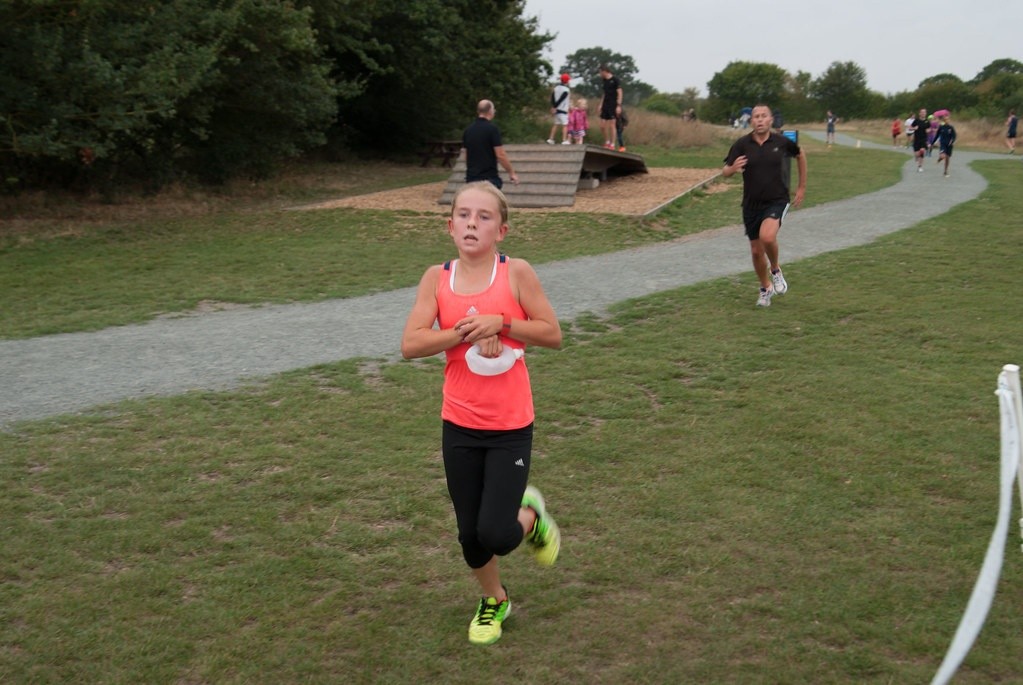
xmin=917 ymin=167 xmax=924 ymax=173
xmin=547 ymin=140 xmax=554 ymax=144
xmin=604 ymin=143 xmax=616 ymax=150
xmin=943 ymin=172 xmax=949 ymax=178
xmin=562 ymin=140 xmax=571 ymax=144
xmin=1009 ymin=148 xmax=1014 ymax=155
xmin=618 ymin=147 xmax=626 ymax=152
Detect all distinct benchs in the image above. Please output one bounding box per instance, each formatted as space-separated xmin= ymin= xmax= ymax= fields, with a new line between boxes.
xmin=418 ymin=150 xmax=460 ymax=169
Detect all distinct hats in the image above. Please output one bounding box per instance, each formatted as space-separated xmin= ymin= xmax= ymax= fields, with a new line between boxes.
xmin=560 ymin=74 xmax=571 ymax=84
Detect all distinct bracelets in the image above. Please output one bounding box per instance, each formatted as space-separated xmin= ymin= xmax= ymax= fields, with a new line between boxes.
xmin=500 ymin=312 xmax=511 ymax=337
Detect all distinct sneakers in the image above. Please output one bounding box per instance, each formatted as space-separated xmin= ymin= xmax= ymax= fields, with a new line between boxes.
xmin=756 ymin=283 xmax=775 ymax=306
xmin=468 ymin=584 xmax=511 ymax=644
xmin=770 ymin=268 xmax=787 ymax=295
xmin=521 ymin=485 xmax=561 ymax=568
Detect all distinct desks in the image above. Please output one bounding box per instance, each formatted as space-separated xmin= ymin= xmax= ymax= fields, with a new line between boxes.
xmin=420 ymin=140 xmax=464 ymax=169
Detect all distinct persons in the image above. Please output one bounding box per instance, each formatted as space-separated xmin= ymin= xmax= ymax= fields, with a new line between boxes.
xmin=722 ymin=104 xmax=807 ymax=307
xmin=824 ymin=111 xmax=839 ymax=144
xmin=729 ymin=107 xmax=752 ymax=129
xmin=596 ymin=66 xmax=629 ymax=152
xmin=772 ymin=109 xmax=783 ymax=128
xmin=400 ymin=181 xmax=562 ymax=648
xmin=1004 ymin=109 xmax=1018 ymax=154
xmin=891 ymin=108 xmax=956 ymax=177
xmin=462 ymin=99 xmax=520 ymax=188
xmin=546 ymin=74 xmax=572 ymax=145
xmin=568 ymin=98 xmax=590 ymax=144
